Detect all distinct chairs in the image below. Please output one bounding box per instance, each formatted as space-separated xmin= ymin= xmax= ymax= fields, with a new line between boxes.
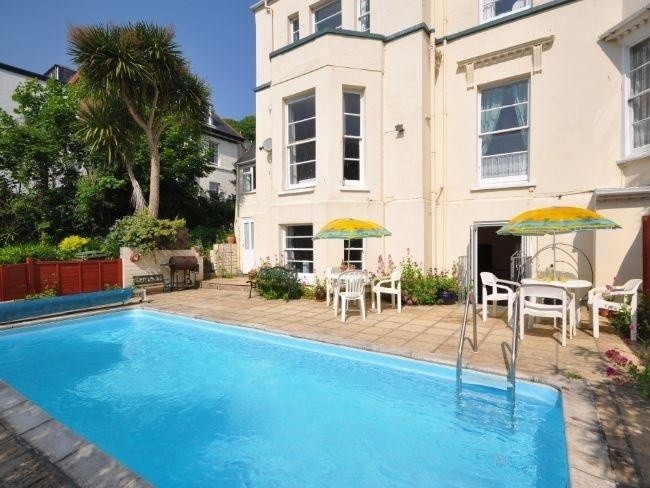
xmin=480 ymin=271 xmax=643 ymax=347
xmin=324 ymin=264 xmax=403 ymax=323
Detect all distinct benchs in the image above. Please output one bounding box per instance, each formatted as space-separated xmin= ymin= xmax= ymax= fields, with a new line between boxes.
xmin=246 ymin=266 xmax=298 ymax=298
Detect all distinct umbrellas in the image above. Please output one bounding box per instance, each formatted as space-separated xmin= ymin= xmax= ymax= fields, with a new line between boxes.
xmin=496 ymin=206 xmax=622 ymax=328
xmin=312 ymin=217 xmax=392 ymax=270
